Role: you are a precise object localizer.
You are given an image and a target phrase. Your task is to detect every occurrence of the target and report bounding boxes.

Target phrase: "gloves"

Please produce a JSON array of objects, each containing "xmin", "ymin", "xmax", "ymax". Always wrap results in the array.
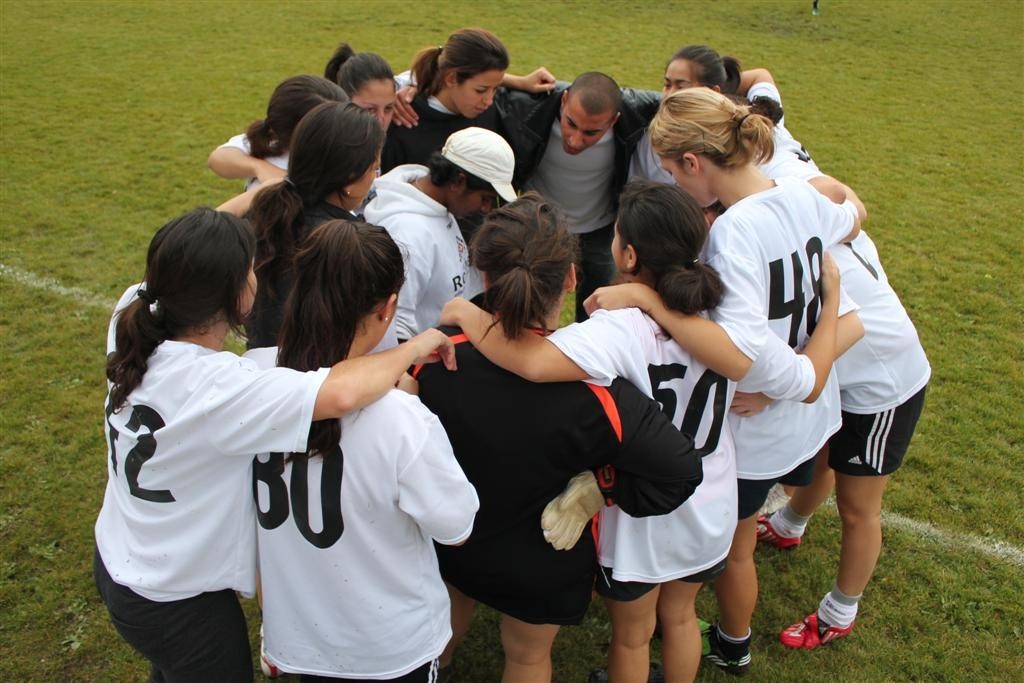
[{"xmin": 539, "ymin": 463, "xmax": 616, "ymax": 550}]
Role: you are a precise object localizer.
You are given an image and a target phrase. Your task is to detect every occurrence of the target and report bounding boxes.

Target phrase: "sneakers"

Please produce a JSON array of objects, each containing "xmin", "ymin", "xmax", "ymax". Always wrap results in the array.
[
  {"xmin": 259, "ymin": 619, "xmax": 286, "ymax": 678},
  {"xmin": 779, "ymin": 610, "xmax": 855, "ymax": 649},
  {"xmin": 756, "ymin": 514, "xmax": 800, "ymax": 549},
  {"xmin": 695, "ymin": 618, "xmax": 752, "ymax": 676},
  {"xmin": 758, "ymin": 483, "xmax": 791, "ymax": 515}
]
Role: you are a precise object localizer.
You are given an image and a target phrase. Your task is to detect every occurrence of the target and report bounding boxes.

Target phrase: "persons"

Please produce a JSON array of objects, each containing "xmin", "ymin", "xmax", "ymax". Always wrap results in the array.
[
  {"xmin": 92, "ymin": 178, "xmax": 457, "ymax": 683},
  {"xmin": 206, "ymin": 28, "xmax": 930, "ymax": 683}
]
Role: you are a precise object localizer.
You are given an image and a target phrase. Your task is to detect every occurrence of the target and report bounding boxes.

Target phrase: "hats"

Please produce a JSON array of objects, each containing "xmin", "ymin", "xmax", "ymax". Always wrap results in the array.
[{"xmin": 442, "ymin": 127, "xmax": 518, "ymax": 203}]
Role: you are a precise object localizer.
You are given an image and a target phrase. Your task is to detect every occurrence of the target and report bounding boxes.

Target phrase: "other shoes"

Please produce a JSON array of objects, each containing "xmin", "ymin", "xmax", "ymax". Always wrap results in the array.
[
  {"xmin": 589, "ymin": 664, "xmax": 609, "ymax": 683},
  {"xmin": 648, "ymin": 662, "xmax": 665, "ymax": 683}
]
